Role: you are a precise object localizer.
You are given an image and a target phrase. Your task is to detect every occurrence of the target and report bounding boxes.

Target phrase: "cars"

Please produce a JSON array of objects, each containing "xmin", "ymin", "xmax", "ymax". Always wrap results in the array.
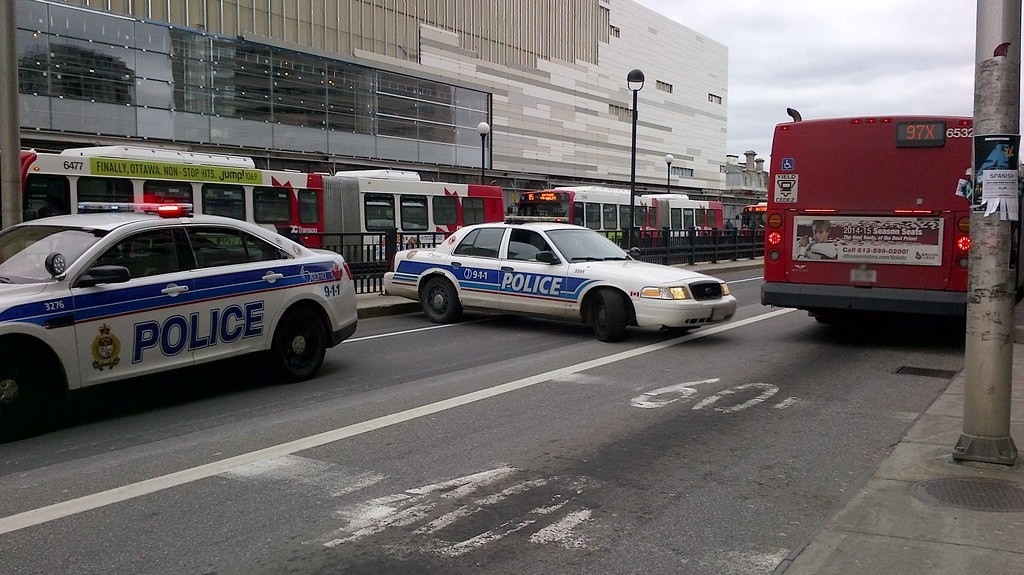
[
  {"xmin": 0, "ymin": 200, "xmax": 360, "ymax": 435},
  {"xmin": 384, "ymin": 216, "xmax": 738, "ymax": 342}
]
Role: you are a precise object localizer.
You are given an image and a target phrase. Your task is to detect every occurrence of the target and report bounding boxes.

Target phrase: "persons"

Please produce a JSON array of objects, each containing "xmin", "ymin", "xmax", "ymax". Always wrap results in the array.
[
  {"xmin": 750, "ymin": 218, "xmax": 761, "ymax": 229},
  {"xmin": 725, "ymin": 219, "xmax": 733, "ymax": 238},
  {"xmin": 797, "ymin": 219, "xmax": 837, "ymax": 260}
]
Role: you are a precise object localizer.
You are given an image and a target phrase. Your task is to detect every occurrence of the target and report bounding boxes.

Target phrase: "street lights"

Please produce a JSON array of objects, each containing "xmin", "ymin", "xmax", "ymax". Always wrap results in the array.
[
  {"xmin": 627, "ymin": 70, "xmax": 645, "ymax": 252},
  {"xmin": 663, "ymin": 153, "xmax": 673, "ymax": 194},
  {"xmin": 477, "ymin": 120, "xmax": 491, "ymax": 185}
]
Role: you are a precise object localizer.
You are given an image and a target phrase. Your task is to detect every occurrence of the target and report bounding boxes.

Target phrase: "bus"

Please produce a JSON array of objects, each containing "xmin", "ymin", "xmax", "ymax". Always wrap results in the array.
[
  {"xmin": 15, "ymin": 142, "xmax": 507, "ymax": 275},
  {"xmin": 736, "ymin": 202, "xmax": 767, "ymax": 246},
  {"xmin": 760, "ymin": 107, "xmax": 1024, "ymax": 332},
  {"xmin": 509, "ymin": 186, "xmax": 724, "ymax": 253}
]
